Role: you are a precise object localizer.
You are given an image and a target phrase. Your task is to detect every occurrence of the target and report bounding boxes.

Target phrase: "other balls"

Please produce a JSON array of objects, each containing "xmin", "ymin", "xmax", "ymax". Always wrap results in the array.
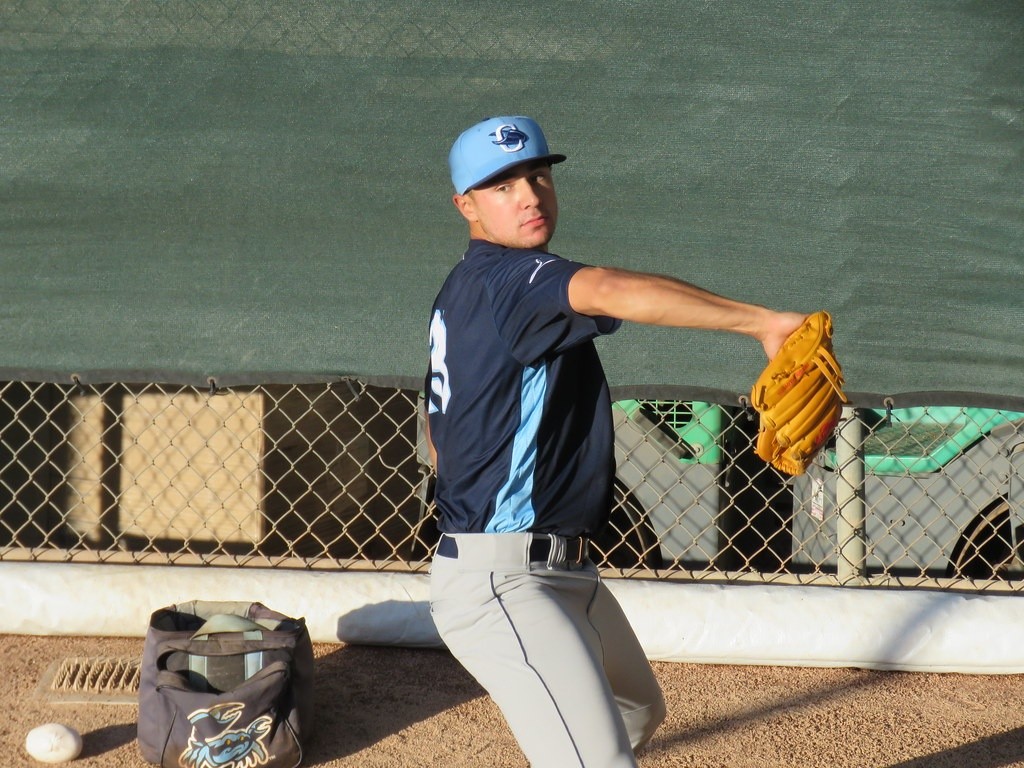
[{"xmin": 24, "ymin": 724, "xmax": 85, "ymax": 763}]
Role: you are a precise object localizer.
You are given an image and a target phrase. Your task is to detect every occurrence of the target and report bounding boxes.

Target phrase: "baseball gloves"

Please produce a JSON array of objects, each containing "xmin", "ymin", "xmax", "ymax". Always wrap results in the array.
[{"xmin": 748, "ymin": 309, "xmax": 848, "ymax": 475}]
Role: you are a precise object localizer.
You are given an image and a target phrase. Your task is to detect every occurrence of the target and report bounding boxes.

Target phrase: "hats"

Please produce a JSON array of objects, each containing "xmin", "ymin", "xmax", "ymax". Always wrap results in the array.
[{"xmin": 447, "ymin": 113, "xmax": 568, "ymax": 196}]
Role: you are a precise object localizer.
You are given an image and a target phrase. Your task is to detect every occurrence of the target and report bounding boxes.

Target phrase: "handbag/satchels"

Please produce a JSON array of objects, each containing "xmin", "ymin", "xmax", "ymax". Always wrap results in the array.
[{"xmin": 136, "ymin": 599, "xmax": 317, "ymax": 768}]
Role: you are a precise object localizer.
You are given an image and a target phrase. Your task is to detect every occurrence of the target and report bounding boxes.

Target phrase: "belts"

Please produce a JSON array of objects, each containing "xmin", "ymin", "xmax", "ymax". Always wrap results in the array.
[{"xmin": 437, "ymin": 533, "xmax": 590, "ymax": 563}]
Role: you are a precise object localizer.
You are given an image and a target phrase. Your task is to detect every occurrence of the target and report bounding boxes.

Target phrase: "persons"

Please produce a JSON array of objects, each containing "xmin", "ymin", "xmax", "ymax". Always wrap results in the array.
[{"xmin": 424, "ymin": 116, "xmax": 847, "ymax": 767}]
